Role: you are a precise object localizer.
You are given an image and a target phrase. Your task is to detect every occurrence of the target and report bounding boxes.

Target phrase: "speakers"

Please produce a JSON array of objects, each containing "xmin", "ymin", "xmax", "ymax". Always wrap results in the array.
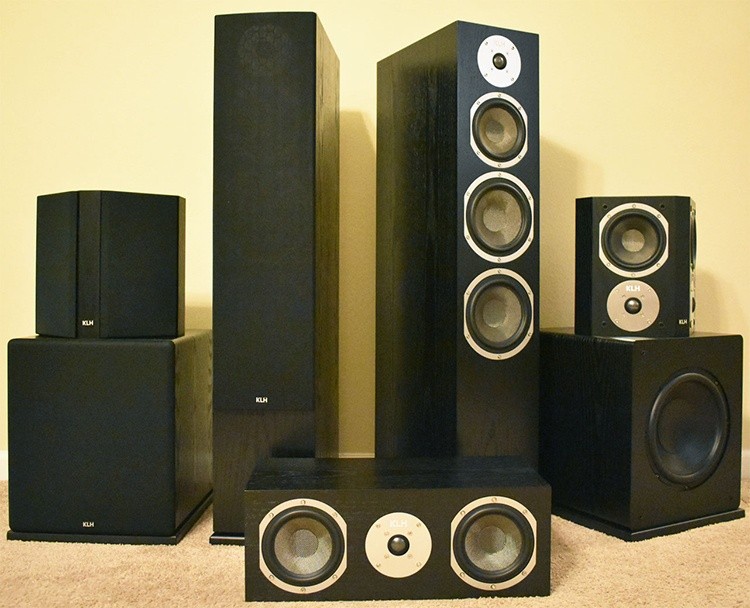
[
  {"xmin": 375, "ymin": 20, "xmax": 540, "ymax": 474},
  {"xmin": 210, "ymin": 10, "xmax": 341, "ymax": 548},
  {"xmin": 574, "ymin": 195, "xmax": 697, "ymax": 338},
  {"xmin": 540, "ymin": 327, "xmax": 745, "ymax": 543},
  {"xmin": 243, "ymin": 457, "xmax": 552, "ymax": 602},
  {"xmin": 7, "ymin": 328, "xmax": 213, "ymax": 545},
  {"xmin": 36, "ymin": 190, "xmax": 186, "ymax": 339}
]
